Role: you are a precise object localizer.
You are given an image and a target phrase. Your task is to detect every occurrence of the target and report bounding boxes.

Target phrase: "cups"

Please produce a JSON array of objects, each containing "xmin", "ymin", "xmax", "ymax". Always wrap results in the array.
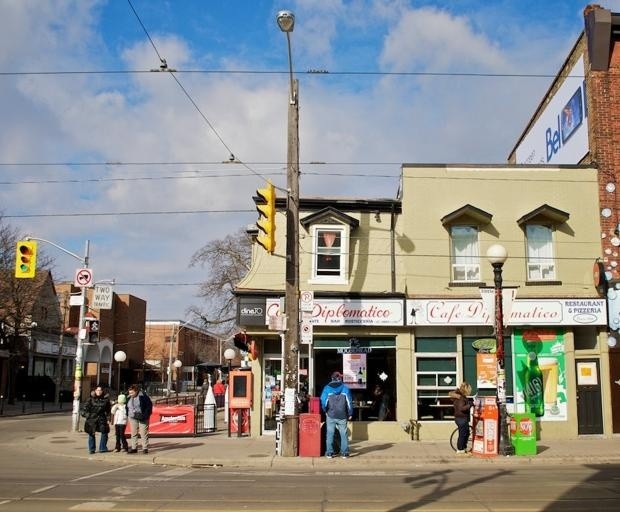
[{"xmin": 535, "ymin": 354, "xmax": 562, "ymax": 410}]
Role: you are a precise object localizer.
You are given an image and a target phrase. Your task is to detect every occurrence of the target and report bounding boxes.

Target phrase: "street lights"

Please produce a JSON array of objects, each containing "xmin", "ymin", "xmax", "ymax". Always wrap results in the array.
[
  {"xmin": 113, "ymin": 350, "xmax": 127, "ymax": 394},
  {"xmin": 487, "ymin": 245, "xmax": 515, "ymax": 456},
  {"xmin": 274, "ymin": 10, "xmax": 300, "ymax": 457},
  {"xmin": 167, "ymin": 315, "xmax": 198, "ymax": 397},
  {"xmin": 175, "ymin": 360, "xmax": 182, "ymax": 402},
  {"xmin": 224, "ymin": 348, "xmax": 235, "ymax": 372}
]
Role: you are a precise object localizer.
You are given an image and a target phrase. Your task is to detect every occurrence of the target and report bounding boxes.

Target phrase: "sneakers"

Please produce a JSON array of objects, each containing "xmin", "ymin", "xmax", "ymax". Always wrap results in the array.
[
  {"xmin": 457, "ymin": 450, "xmax": 471, "ymax": 456},
  {"xmin": 326, "ymin": 452, "xmax": 348, "ymax": 459},
  {"xmin": 114, "ymin": 449, "xmax": 148, "ymax": 454}
]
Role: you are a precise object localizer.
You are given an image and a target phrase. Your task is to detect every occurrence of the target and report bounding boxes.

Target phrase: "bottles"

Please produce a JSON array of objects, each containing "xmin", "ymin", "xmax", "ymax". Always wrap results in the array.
[
  {"xmin": 523, "ymin": 341, "xmax": 544, "ymax": 417},
  {"xmin": 204, "ymin": 381, "xmax": 217, "ymax": 433}
]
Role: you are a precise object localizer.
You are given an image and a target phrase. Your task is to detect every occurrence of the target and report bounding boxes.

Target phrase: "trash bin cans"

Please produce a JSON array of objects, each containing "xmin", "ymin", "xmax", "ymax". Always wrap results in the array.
[{"xmin": 280, "ymin": 413, "xmax": 299, "ymax": 459}]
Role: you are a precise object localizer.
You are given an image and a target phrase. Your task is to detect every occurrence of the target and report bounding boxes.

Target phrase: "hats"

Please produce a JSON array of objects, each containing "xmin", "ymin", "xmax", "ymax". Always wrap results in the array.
[
  {"xmin": 332, "ymin": 372, "xmax": 344, "ymax": 380},
  {"xmin": 118, "ymin": 394, "xmax": 127, "ymax": 403}
]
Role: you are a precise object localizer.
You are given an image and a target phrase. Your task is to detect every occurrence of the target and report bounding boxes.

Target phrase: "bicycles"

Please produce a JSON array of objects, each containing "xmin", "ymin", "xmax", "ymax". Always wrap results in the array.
[{"xmin": 451, "ymin": 424, "xmax": 515, "ymax": 454}]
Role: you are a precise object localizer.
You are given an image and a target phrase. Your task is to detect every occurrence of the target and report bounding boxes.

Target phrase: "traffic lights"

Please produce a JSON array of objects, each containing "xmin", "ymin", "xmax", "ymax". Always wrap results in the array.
[
  {"xmin": 90, "ymin": 321, "xmax": 100, "ymax": 344},
  {"xmin": 256, "ymin": 183, "xmax": 275, "ymax": 257},
  {"xmin": 15, "ymin": 240, "xmax": 36, "ymax": 279}
]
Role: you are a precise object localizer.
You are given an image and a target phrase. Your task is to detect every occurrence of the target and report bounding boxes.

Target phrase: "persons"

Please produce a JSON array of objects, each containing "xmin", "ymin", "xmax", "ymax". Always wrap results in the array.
[
  {"xmin": 213, "ymin": 379, "xmax": 225, "ymax": 397},
  {"xmin": 80, "ymin": 384, "xmax": 114, "ymax": 454},
  {"xmin": 85, "ymin": 388, "xmax": 96, "ymax": 403},
  {"xmin": 200, "ymin": 379, "xmax": 209, "ymax": 397},
  {"xmin": 453, "ymin": 381, "xmax": 478, "ymax": 455},
  {"xmin": 361, "ymin": 380, "xmax": 386, "ymax": 420},
  {"xmin": 320, "ymin": 371, "xmax": 355, "ymax": 458},
  {"xmin": 126, "ymin": 384, "xmax": 154, "ymax": 454},
  {"xmin": 110, "ymin": 394, "xmax": 130, "ymax": 453}
]
